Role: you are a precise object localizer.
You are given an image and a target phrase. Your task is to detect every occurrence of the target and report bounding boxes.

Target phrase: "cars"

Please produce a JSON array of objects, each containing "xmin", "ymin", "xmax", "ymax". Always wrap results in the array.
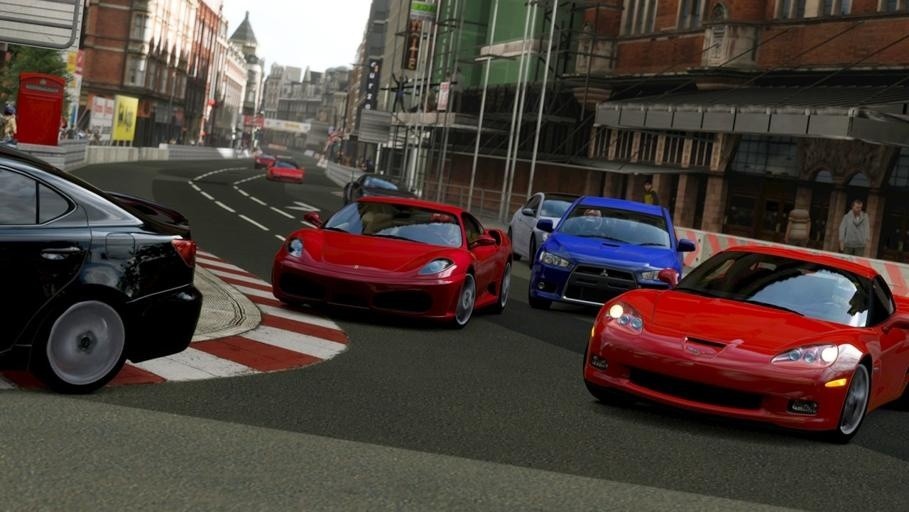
[
  {"xmin": 0, "ymin": 140, "xmax": 203, "ymax": 395},
  {"xmin": 508, "ymin": 192, "xmax": 697, "ymax": 311}
]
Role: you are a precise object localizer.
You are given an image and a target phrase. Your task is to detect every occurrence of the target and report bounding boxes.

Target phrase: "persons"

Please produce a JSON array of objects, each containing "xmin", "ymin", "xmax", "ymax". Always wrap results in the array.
[
  {"xmin": 642, "ymin": 181, "xmax": 659, "ymax": 206},
  {"xmin": 569, "ymin": 208, "xmax": 608, "ymax": 234},
  {"xmin": 784, "ymin": 197, "xmax": 812, "ymax": 245},
  {"xmin": 59, "ymin": 125, "xmax": 91, "ymax": 141},
  {"xmin": 838, "ymin": 197, "xmax": 870, "ymax": 257},
  {"xmin": 0, "ymin": 104, "xmax": 17, "ymax": 145},
  {"xmin": 392, "ymin": 72, "xmax": 408, "ymax": 112}
]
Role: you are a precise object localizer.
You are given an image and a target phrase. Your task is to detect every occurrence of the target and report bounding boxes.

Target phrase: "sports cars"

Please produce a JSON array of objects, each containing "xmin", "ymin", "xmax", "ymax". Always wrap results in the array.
[
  {"xmin": 583, "ymin": 245, "xmax": 909, "ymax": 445},
  {"xmin": 271, "ymin": 173, "xmax": 514, "ymax": 330},
  {"xmin": 253, "ymin": 151, "xmax": 306, "ymax": 183}
]
